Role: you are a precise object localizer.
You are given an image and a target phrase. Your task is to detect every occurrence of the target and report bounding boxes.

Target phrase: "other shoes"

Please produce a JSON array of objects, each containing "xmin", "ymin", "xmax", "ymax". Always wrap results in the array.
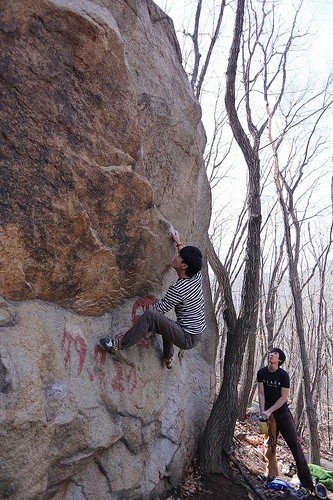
[
  {"xmin": 99, "ymin": 337, "xmax": 119, "ymax": 357},
  {"xmin": 298, "ymin": 486, "xmax": 312, "ymax": 497},
  {"xmin": 165, "ymin": 357, "xmax": 173, "ymax": 369}
]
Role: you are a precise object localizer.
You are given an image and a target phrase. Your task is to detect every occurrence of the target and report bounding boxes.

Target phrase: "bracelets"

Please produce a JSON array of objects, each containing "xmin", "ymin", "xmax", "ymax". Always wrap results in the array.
[{"xmin": 176, "ymin": 244, "xmax": 184, "ymax": 247}]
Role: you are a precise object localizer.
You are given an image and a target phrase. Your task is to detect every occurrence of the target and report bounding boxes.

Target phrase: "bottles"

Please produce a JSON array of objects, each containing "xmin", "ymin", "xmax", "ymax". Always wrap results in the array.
[{"xmin": 258, "ymin": 415, "xmax": 269, "ymax": 434}]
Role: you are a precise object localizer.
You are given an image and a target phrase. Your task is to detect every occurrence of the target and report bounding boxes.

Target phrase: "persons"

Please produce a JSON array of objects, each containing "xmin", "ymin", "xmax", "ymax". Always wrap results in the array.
[
  {"xmin": 258, "ymin": 348, "xmax": 314, "ymax": 489},
  {"xmin": 98, "ymin": 230, "xmax": 207, "ymax": 370}
]
